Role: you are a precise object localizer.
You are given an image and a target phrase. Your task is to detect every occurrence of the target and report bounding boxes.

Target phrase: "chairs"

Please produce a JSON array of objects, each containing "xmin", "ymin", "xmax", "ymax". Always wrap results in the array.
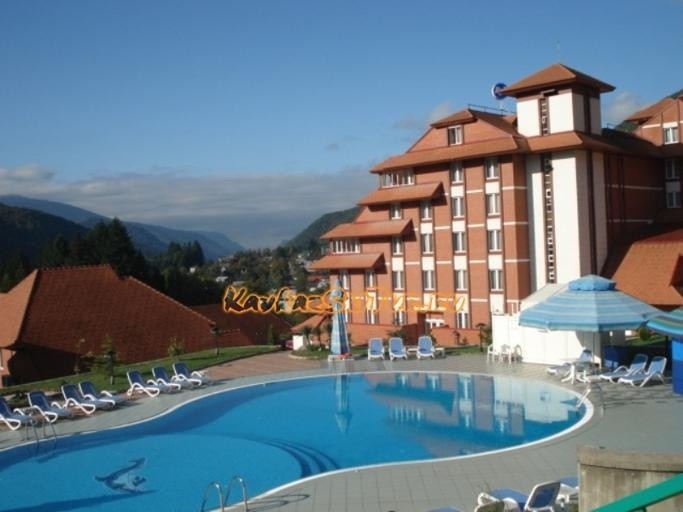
[
  {"xmin": 546, "ymin": 349, "xmax": 668, "ymax": 388},
  {"xmin": 426, "ymin": 475, "xmax": 580, "ymax": 512},
  {"xmin": 487, "ymin": 342, "xmax": 522, "ymax": 364},
  {"xmin": 0, "ymin": 362, "xmax": 212, "ymax": 434},
  {"xmin": 365, "ymin": 335, "xmax": 436, "ymax": 362}
]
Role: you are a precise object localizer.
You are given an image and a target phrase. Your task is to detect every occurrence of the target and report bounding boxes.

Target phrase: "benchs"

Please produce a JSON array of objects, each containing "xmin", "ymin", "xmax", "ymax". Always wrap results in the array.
[{"xmin": 406, "ymin": 348, "xmax": 445, "ymax": 356}]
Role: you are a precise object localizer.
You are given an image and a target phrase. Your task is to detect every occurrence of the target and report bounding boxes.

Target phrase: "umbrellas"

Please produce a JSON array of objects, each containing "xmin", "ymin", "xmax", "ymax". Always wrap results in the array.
[
  {"xmin": 332, "ymin": 375, "xmax": 353, "ymax": 438},
  {"xmin": 515, "ymin": 273, "xmax": 659, "ymax": 369},
  {"xmin": 329, "ymin": 280, "xmax": 351, "ymax": 358},
  {"xmin": 644, "ymin": 303, "xmax": 683, "ymax": 341}
]
